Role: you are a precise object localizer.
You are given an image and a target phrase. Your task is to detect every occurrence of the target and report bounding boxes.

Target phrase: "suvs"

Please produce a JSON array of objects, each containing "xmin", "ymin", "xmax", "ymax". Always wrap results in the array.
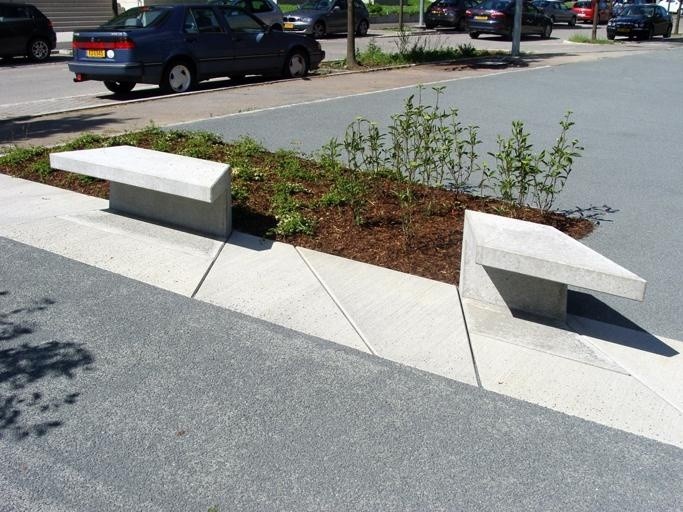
[{"xmin": 68, "ymin": 1, "xmax": 325, "ymax": 94}]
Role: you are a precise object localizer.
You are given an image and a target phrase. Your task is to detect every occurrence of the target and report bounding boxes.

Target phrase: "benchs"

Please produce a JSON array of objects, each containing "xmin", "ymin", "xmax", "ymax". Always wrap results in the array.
[
  {"xmin": 456, "ymin": 206, "xmax": 647, "ymax": 328},
  {"xmin": 46, "ymin": 144, "xmax": 234, "ymax": 239}
]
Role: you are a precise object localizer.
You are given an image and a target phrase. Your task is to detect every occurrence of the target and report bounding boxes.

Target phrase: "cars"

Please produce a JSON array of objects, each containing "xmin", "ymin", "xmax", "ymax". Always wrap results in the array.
[
  {"xmin": 424, "ymin": 0, "xmax": 683, "ymax": 41},
  {"xmin": 0, "ymin": 1, "xmax": 59, "ymax": 63},
  {"xmin": 282, "ymin": 0, "xmax": 369, "ymax": 39},
  {"xmin": 184, "ymin": 1, "xmax": 284, "ymax": 33}
]
